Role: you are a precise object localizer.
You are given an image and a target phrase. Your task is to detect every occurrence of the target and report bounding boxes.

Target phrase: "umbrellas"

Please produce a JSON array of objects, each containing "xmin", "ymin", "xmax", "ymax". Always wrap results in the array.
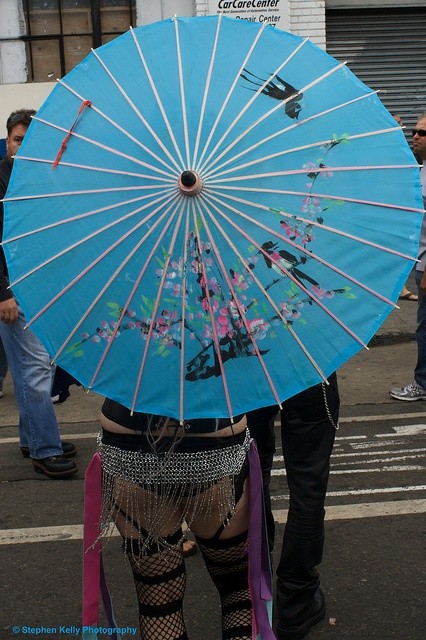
[{"xmin": 0, "ymin": 14, "xmax": 426, "ymax": 426}]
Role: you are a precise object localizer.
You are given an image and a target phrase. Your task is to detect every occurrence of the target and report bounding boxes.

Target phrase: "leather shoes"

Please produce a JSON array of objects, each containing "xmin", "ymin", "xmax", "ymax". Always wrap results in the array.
[
  {"xmin": 22, "ymin": 442, "xmax": 75, "ymax": 456},
  {"xmin": 275, "ymin": 588, "xmax": 325, "ymax": 640}
]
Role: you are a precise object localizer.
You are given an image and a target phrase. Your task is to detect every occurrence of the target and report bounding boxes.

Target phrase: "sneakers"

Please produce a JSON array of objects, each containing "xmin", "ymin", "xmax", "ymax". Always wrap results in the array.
[
  {"xmin": 33, "ymin": 457, "xmax": 76, "ymax": 474},
  {"xmin": 390, "ymin": 380, "xmax": 426, "ymax": 401}
]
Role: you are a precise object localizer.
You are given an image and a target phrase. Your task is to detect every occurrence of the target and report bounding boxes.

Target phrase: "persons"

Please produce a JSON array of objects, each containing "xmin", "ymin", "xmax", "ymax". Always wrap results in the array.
[
  {"xmin": 2, "ymin": 109, "xmax": 77, "ymax": 476},
  {"xmin": 389, "ymin": 115, "xmax": 426, "ymax": 401},
  {"xmin": 248, "ymin": 372, "xmax": 339, "ymax": 640},
  {"xmin": 94, "ymin": 397, "xmax": 261, "ymax": 640}
]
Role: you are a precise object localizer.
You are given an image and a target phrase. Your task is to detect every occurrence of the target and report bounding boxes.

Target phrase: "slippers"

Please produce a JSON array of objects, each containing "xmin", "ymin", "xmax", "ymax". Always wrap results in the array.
[{"xmin": 399, "ymin": 292, "xmax": 418, "ymax": 301}]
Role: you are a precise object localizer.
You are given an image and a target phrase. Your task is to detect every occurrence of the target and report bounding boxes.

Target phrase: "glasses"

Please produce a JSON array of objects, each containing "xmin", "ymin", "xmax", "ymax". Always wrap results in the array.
[{"xmin": 412, "ymin": 130, "xmax": 426, "ymax": 136}]
